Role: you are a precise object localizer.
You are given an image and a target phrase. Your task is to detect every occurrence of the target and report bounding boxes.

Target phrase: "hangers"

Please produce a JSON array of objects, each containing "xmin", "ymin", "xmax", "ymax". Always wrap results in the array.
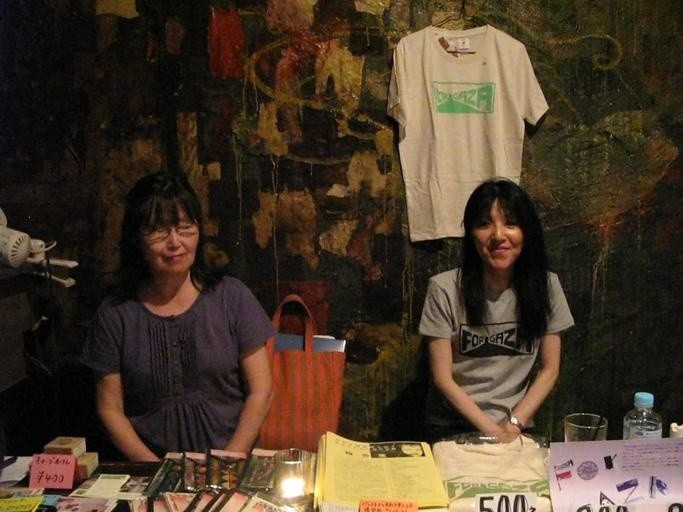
[{"xmin": 395, "ymin": 1, "xmax": 525, "ymax": 56}]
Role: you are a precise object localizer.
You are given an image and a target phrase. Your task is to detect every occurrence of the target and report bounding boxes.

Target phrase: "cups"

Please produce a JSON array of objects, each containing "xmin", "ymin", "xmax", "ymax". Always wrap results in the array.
[
  {"xmin": 272, "ymin": 447, "xmax": 312, "ymax": 511},
  {"xmin": 564, "ymin": 413, "xmax": 608, "ymax": 443}
]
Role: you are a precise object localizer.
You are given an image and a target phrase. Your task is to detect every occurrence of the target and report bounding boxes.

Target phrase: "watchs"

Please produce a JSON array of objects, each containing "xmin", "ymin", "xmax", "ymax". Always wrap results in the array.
[{"xmin": 506, "ymin": 415, "xmax": 525, "ymax": 432}]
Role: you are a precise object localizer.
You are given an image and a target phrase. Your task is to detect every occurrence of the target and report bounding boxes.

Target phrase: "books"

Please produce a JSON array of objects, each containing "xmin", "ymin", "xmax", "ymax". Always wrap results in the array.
[{"xmin": 310, "ymin": 430, "xmax": 450, "ymax": 512}]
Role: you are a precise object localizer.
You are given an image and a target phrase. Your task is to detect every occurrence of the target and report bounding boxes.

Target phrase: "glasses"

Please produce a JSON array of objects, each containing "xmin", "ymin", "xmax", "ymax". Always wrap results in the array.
[{"xmin": 140, "ymin": 221, "xmax": 198, "ymax": 245}]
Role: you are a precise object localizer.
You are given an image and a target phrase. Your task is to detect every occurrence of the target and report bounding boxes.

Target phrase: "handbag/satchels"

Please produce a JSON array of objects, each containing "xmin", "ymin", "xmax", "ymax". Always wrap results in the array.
[{"xmin": 259, "ymin": 294, "xmax": 347, "ymax": 453}]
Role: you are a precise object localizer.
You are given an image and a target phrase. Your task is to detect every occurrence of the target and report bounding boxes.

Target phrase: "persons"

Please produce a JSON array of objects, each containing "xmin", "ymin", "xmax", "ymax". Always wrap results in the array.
[
  {"xmin": 84, "ymin": 168, "xmax": 275, "ymax": 463},
  {"xmin": 414, "ymin": 177, "xmax": 577, "ymax": 443}
]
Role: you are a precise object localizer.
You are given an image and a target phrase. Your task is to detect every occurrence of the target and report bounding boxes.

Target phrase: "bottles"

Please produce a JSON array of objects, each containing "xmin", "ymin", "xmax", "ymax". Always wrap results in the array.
[{"xmin": 623, "ymin": 392, "xmax": 663, "ymax": 440}]
